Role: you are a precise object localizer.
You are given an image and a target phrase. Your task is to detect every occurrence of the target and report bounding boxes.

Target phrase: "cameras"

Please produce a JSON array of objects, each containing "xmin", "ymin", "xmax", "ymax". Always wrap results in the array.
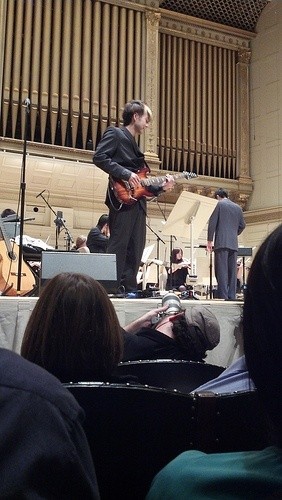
[{"xmin": 150, "ymin": 293, "xmax": 182, "ymax": 325}]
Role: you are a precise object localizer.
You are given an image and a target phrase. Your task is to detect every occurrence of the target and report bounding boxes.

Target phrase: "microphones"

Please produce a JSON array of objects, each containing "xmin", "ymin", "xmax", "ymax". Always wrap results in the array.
[
  {"xmin": 24, "ymin": 98, "xmax": 31, "ymax": 113},
  {"xmin": 56, "ymin": 211, "xmax": 63, "ymax": 235},
  {"xmin": 149, "ymin": 197, "xmax": 157, "ymax": 203},
  {"xmin": 36, "ymin": 190, "xmax": 45, "ymax": 198}
]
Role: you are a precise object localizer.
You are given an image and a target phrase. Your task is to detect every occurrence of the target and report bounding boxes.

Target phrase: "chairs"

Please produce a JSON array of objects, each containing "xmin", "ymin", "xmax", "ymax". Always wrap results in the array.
[{"xmin": 62, "ymin": 359, "xmax": 275, "ymax": 500}]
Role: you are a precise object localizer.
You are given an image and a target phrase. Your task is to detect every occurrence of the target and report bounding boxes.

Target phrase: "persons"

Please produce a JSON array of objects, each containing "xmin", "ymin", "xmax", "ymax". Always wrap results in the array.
[
  {"xmin": 207, "ymin": 189, "xmax": 246, "ymax": 299},
  {"xmin": 0, "ymin": 209, "xmax": 282, "ymax": 500},
  {"xmin": 93, "ymin": 100, "xmax": 174, "ymax": 298}
]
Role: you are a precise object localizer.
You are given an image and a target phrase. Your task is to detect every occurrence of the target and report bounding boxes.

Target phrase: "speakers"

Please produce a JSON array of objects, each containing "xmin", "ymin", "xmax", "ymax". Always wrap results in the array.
[{"xmin": 39, "ymin": 251, "xmax": 118, "ymax": 295}]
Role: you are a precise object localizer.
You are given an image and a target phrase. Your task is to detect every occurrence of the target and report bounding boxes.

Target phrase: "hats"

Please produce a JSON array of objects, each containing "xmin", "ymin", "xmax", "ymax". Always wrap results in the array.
[{"xmin": 184, "ymin": 305, "xmax": 221, "ymax": 351}]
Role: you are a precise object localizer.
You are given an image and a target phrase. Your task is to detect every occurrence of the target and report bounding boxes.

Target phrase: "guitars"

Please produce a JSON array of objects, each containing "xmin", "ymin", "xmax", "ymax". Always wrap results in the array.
[
  {"xmin": 108, "ymin": 167, "xmax": 198, "ymax": 208},
  {"xmin": 0, "ymin": 215, "xmax": 36, "ymax": 296}
]
